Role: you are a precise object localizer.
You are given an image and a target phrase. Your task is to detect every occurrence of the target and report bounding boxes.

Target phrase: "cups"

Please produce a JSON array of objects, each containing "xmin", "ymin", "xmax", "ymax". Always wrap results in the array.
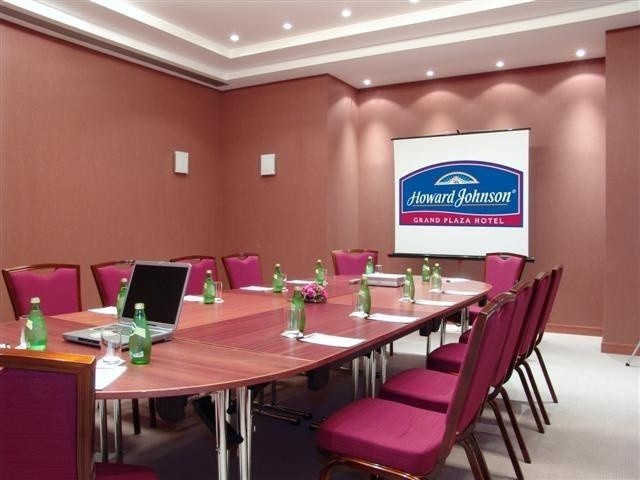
[
  {"xmin": 323, "ymin": 268, "xmax": 328, "ymax": 285},
  {"xmin": 281, "ymin": 274, "xmax": 287, "ymax": 291},
  {"xmin": 400, "ymin": 280, "xmax": 412, "ymax": 304},
  {"xmin": 375, "ymin": 264, "xmax": 383, "ymax": 275},
  {"xmin": 429, "ymin": 276, "xmax": 442, "ymax": 292},
  {"xmin": 286, "ymin": 304, "xmax": 300, "ymax": 335},
  {"xmin": 214, "ymin": 282, "xmax": 223, "ymax": 301},
  {"xmin": 101, "ymin": 328, "xmax": 123, "ymax": 365},
  {"xmin": 352, "ymin": 290, "xmax": 365, "ymax": 315},
  {"xmin": 18, "ymin": 315, "xmax": 28, "ymax": 348}
]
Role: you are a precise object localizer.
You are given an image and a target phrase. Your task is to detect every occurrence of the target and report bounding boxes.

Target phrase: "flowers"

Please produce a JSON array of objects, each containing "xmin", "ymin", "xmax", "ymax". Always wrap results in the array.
[{"xmin": 300, "ymin": 282, "xmax": 329, "ymax": 303}]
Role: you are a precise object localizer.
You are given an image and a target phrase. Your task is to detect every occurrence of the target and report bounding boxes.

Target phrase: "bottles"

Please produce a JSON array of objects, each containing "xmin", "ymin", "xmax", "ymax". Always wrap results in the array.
[
  {"xmin": 272, "ymin": 264, "xmax": 282, "ymax": 293},
  {"xmin": 291, "ymin": 286, "xmax": 305, "ymax": 333},
  {"xmin": 203, "ymin": 270, "xmax": 215, "ymax": 304},
  {"xmin": 25, "ymin": 297, "xmax": 47, "ymax": 352},
  {"xmin": 117, "ymin": 277, "xmax": 128, "ymax": 318},
  {"xmin": 315, "ymin": 259, "xmax": 323, "ymax": 285},
  {"xmin": 405, "ymin": 268, "xmax": 415, "ymax": 303},
  {"xmin": 432, "ymin": 262, "xmax": 441, "ymax": 289},
  {"xmin": 422, "ymin": 257, "xmax": 430, "ymax": 283},
  {"xmin": 365, "ymin": 255, "xmax": 374, "ymax": 274},
  {"xmin": 128, "ymin": 302, "xmax": 152, "ymax": 364},
  {"xmin": 358, "ymin": 274, "xmax": 371, "ymax": 315}
]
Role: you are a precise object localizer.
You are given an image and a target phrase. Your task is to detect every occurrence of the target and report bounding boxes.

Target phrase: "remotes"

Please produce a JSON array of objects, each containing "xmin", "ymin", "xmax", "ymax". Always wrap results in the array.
[{"xmin": 349, "ymin": 279, "xmax": 361, "ymax": 284}]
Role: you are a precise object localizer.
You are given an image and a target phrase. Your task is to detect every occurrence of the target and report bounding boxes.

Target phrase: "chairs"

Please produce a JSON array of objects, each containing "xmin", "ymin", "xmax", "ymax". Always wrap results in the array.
[
  {"xmin": 438, "ymin": 252, "xmax": 527, "ymax": 346},
  {"xmin": 424, "ymin": 272, "xmax": 553, "ymax": 463},
  {"xmin": 316, "ymin": 292, "xmax": 517, "ymax": 480},
  {"xmin": 331, "ymin": 248, "xmax": 380, "ymax": 274},
  {"xmin": 221, "ymin": 251, "xmax": 264, "ymax": 289},
  {"xmin": 2, "ymin": 262, "xmax": 126, "ymax": 466},
  {"xmin": 170, "ymin": 255, "xmax": 218, "ymax": 295},
  {"xmin": 380, "ymin": 278, "xmax": 536, "ymax": 479},
  {"xmin": 90, "ymin": 258, "xmax": 164, "ymax": 434},
  {"xmin": 460, "ymin": 265, "xmax": 564, "ymax": 424},
  {"xmin": 0, "ymin": 349, "xmax": 158, "ymax": 480}
]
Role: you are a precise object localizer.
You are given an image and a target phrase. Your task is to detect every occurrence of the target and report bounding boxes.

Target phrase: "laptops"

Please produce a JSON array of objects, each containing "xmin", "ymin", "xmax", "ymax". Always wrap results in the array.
[{"xmin": 62, "ymin": 260, "xmax": 192, "ymax": 353}]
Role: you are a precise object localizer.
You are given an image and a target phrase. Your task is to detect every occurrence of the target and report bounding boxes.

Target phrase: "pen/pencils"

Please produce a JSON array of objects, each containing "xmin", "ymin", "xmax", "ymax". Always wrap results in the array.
[{"xmin": 298, "ymin": 334, "xmax": 313, "ymax": 339}]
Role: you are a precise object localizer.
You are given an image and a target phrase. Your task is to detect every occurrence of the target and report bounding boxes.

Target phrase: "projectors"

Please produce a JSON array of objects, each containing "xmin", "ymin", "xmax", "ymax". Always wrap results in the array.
[{"xmin": 366, "ymin": 272, "xmax": 407, "ymax": 288}]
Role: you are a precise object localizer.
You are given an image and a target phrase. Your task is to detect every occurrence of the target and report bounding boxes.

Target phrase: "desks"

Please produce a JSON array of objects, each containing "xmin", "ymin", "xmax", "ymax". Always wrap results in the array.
[{"xmin": 0, "ymin": 276, "xmax": 492, "ymax": 480}]
